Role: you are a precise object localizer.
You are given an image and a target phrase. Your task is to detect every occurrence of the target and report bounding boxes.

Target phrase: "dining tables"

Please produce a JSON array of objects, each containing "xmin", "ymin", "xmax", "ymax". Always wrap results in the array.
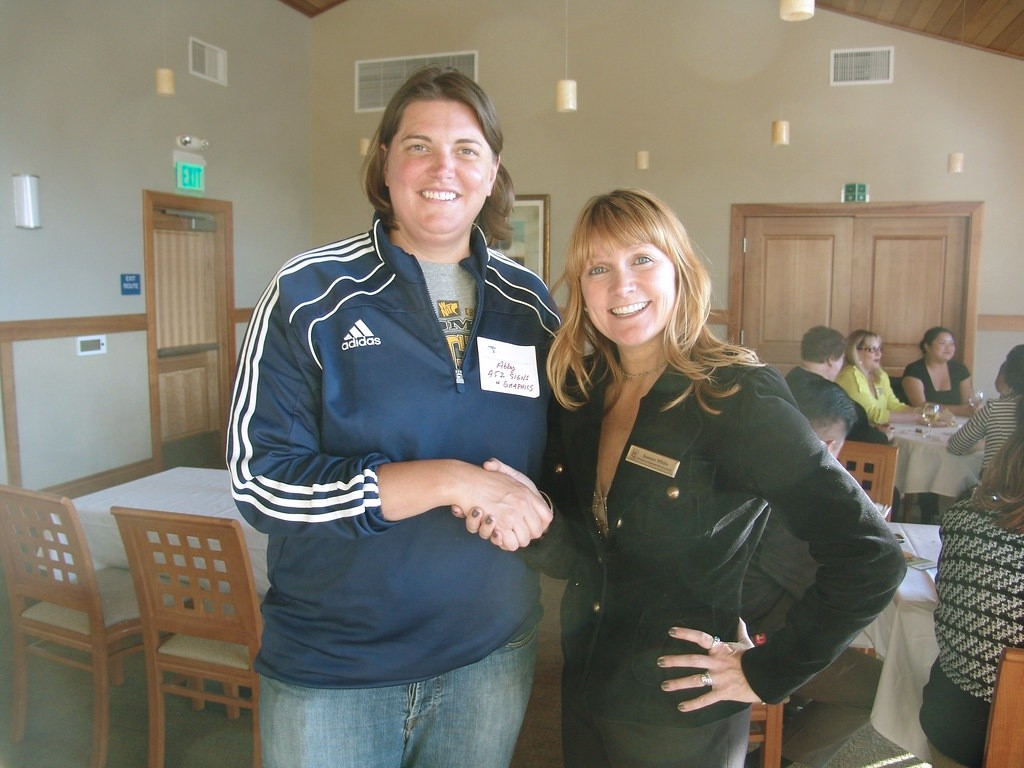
[
  {"xmin": 880, "ymin": 414, "xmax": 987, "ymax": 522},
  {"xmin": 36, "ymin": 466, "xmax": 273, "ymax": 597},
  {"xmin": 826, "ymin": 523, "xmax": 942, "ymax": 760}
]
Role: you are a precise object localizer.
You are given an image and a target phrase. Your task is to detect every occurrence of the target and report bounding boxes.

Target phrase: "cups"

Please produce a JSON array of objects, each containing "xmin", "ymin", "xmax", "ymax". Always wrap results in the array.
[{"xmin": 969, "ymin": 390, "xmax": 984, "ymax": 409}]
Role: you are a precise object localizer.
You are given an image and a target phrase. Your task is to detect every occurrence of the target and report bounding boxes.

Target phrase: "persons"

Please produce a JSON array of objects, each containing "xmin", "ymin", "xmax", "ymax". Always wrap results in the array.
[
  {"xmin": 741, "ymin": 366, "xmax": 885, "ymax": 768},
  {"xmin": 901, "ymin": 327, "xmax": 974, "ymax": 524},
  {"xmin": 785, "ymin": 326, "xmax": 899, "ymax": 521},
  {"xmin": 918, "ymin": 398, "xmax": 1024, "ymax": 768},
  {"xmin": 835, "ymin": 330, "xmax": 954, "ymax": 427},
  {"xmin": 225, "ymin": 62, "xmax": 560, "ymax": 768},
  {"xmin": 949, "ymin": 344, "xmax": 1024, "ymax": 502},
  {"xmin": 453, "ymin": 189, "xmax": 907, "ymax": 768}
]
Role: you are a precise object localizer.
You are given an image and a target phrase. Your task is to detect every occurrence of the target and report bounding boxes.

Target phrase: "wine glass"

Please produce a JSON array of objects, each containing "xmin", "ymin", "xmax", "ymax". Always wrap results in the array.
[{"xmin": 922, "ymin": 402, "xmax": 940, "ymax": 440}]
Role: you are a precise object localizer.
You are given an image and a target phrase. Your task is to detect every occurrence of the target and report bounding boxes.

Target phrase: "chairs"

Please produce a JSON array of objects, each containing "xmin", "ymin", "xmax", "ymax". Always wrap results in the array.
[
  {"xmin": 110, "ymin": 504, "xmax": 264, "ymax": 768},
  {"xmin": 746, "ymin": 439, "xmax": 1024, "ymax": 768},
  {"xmin": 0, "ymin": 485, "xmax": 206, "ymax": 768}
]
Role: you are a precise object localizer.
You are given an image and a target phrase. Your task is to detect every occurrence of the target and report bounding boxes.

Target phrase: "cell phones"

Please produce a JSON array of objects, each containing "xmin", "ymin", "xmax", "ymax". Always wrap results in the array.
[{"xmin": 895, "ymin": 534, "xmax": 906, "ymax": 543}]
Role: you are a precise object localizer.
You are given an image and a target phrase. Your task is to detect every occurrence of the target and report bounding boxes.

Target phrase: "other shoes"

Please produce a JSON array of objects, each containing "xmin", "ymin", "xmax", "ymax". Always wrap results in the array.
[{"xmin": 743, "ymin": 746, "xmax": 814, "ymax": 768}]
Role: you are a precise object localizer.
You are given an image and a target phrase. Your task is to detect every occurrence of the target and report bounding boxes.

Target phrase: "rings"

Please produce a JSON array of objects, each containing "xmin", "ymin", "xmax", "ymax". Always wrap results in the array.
[
  {"xmin": 701, "ymin": 672, "xmax": 712, "ymax": 686},
  {"xmin": 711, "ymin": 636, "xmax": 720, "ymax": 647}
]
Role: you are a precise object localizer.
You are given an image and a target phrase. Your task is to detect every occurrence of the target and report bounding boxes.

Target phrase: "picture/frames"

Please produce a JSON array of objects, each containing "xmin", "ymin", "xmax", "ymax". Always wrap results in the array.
[{"xmin": 489, "ymin": 193, "xmax": 550, "ymax": 290}]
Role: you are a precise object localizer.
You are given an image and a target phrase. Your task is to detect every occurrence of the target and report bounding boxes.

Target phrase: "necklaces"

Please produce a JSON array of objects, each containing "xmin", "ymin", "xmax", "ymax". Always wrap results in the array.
[{"xmin": 620, "ymin": 360, "xmax": 670, "ymax": 379}]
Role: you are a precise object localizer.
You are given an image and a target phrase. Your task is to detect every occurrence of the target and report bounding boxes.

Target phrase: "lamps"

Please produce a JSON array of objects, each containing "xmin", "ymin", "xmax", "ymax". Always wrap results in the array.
[
  {"xmin": 555, "ymin": 0, "xmax": 576, "ymax": 112},
  {"xmin": 12, "ymin": 174, "xmax": 42, "ymax": 227},
  {"xmin": 779, "ymin": 0, "xmax": 815, "ymax": 21},
  {"xmin": 178, "ymin": 136, "xmax": 210, "ymax": 151},
  {"xmin": 638, "ymin": 150, "xmax": 649, "ymax": 169},
  {"xmin": 772, "ymin": 81, "xmax": 790, "ymax": 144},
  {"xmin": 156, "ymin": 0, "xmax": 174, "ymax": 96},
  {"xmin": 949, "ymin": 0, "xmax": 965, "ymax": 174},
  {"xmin": 359, "ymin": 139, "xmax": 370, "ymax": 156}
]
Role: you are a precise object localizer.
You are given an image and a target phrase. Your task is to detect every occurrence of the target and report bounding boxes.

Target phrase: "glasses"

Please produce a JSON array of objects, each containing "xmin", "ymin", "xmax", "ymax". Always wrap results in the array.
[{"xmin": 857, "ymin": 347, "xmax": 882, "ymax": 353}]
